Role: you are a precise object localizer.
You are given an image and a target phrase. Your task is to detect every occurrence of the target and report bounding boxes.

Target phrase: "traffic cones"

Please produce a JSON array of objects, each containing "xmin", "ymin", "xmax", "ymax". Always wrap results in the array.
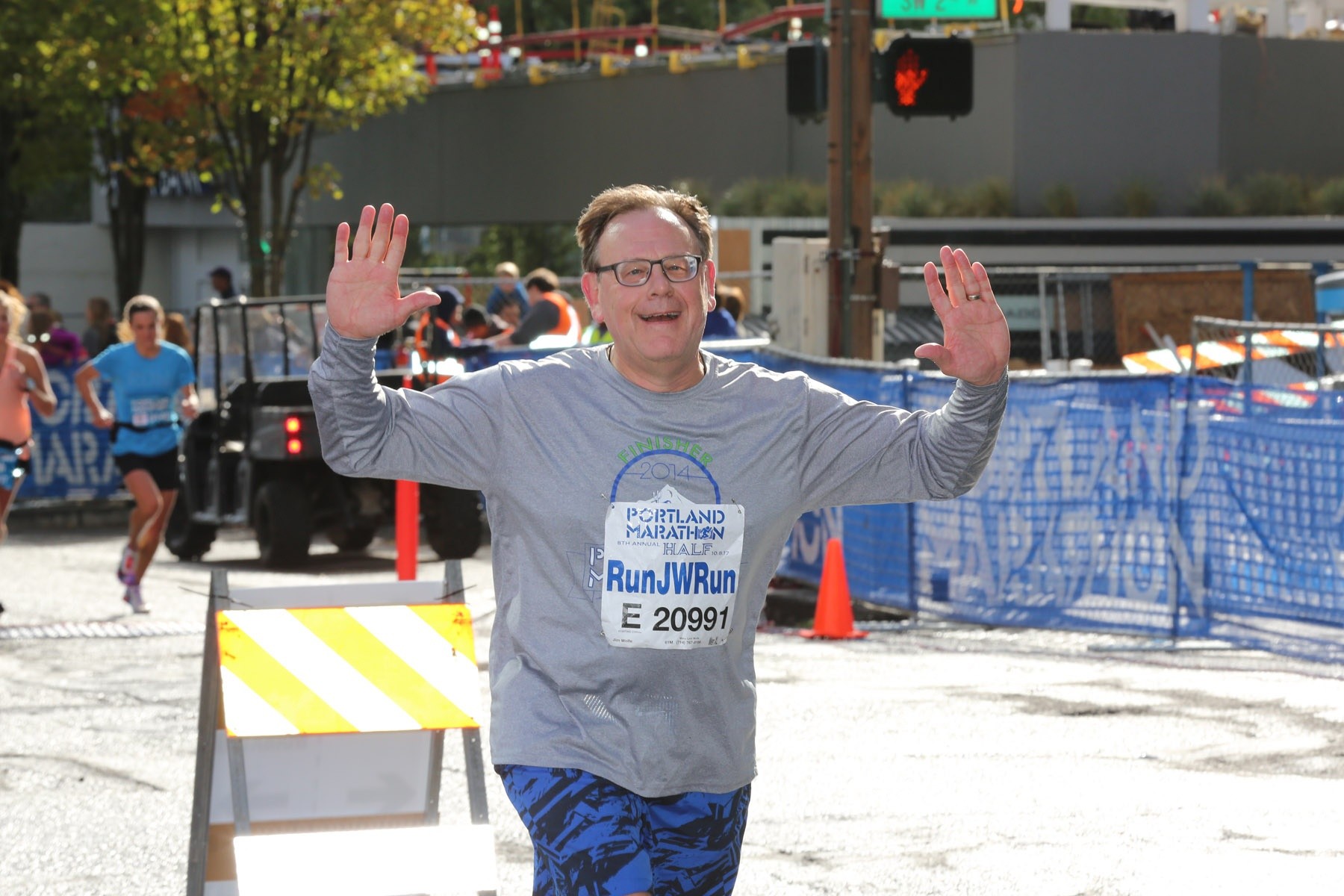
[{"xmin": 798, "ymin": 539, "xmax": 871, "ymax": 641}]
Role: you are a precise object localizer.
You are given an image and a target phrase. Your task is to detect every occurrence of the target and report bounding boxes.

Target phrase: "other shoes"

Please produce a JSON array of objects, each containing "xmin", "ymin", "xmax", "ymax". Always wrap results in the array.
[
  {"xmin": 123, "ymin": 584, "xmax": 151, "ymax": 613},
  {"xmin": 117, "ymin": 547, "xmax": 140, "ymax": 585}
]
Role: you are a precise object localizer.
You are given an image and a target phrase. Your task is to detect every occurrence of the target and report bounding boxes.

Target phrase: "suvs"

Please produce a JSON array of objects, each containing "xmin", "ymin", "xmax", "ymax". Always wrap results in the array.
[{"xmin": 163, "ymin": 295, "xmax": 483, "ymax": 568}]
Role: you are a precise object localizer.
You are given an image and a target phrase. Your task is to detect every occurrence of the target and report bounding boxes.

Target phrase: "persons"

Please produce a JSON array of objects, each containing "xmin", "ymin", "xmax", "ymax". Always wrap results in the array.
[
  {"xmin": 0, "ymin": 266, "xmax": 266, "ymax": 616},
  {"xmin": 308, "ymin": 184, "xmax": 1010, "ymax": 896},
  {"xmin": 398, "ymin": 262, "xmax": 747, "ymax": 395}
]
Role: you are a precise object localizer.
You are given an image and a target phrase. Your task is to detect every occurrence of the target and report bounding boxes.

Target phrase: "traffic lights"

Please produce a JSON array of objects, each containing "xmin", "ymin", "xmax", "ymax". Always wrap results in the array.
[
  {"xmin": 782, "ymin": 38, "xmax": 831, "ymax": 124},
  {"xmin": 1006, "ymin": 0, "xmax": 1046, "ymax": 18},
  {"xmin": 881, "ymin": 31, "xmax": 975, "ymax": 123}
]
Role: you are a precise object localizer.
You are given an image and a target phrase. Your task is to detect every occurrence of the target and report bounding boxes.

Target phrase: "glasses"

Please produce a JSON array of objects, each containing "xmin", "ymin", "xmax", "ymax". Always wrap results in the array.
[{"xmin": 596, "ymin": 254, "xmax": 702, "ymax": 286}]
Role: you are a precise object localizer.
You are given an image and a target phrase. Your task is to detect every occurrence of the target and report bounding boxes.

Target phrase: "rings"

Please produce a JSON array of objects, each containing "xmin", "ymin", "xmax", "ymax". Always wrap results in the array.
[{"xmin": 966, "ymin": 293, "xmax": 981, "ymax": 300}]
